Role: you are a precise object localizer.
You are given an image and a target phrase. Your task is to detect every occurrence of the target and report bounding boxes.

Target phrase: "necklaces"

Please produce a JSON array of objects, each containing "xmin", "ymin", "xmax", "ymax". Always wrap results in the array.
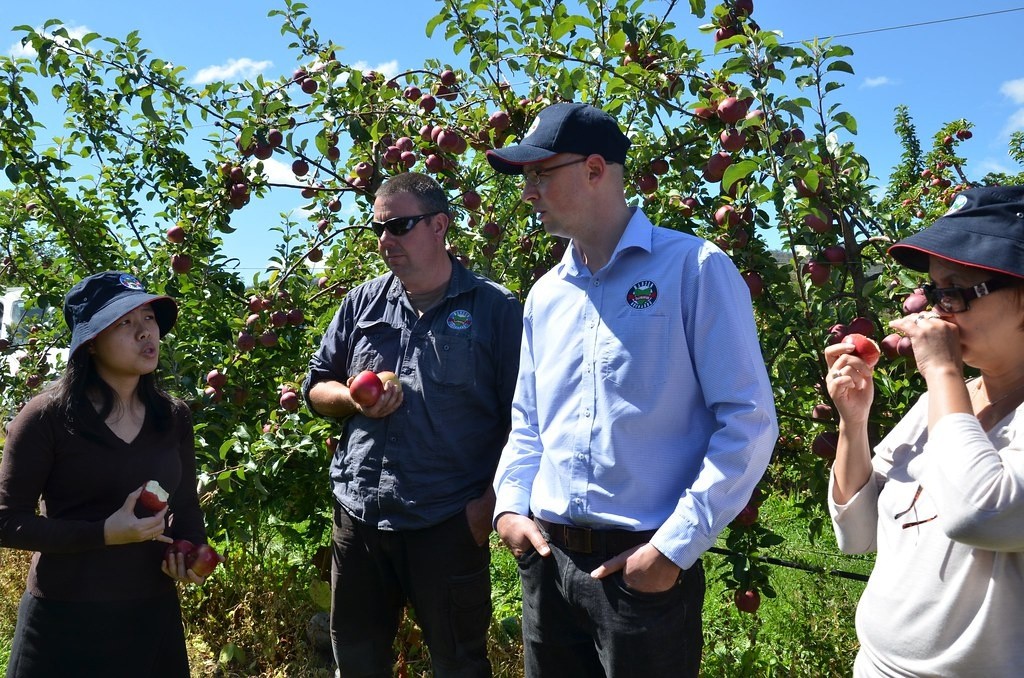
[{"xmin": 981, "ymin": 383, "xmax": 1024, "ymax": 406}]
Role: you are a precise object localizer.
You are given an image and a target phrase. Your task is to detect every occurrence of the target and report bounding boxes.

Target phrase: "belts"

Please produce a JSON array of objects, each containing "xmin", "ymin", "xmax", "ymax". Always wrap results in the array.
[{"xmin": 532, "ymin": 516, "xmax": 658, "ymax": 556}]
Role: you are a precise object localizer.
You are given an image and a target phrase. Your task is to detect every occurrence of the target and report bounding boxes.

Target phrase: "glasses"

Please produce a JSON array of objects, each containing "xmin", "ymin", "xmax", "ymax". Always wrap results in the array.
[
  {"xmin": 522, "ymin": 157, "xmax": 614, "ymax": 186},
  {"xmin": 923, "ymin": 274, "xmax": 1015, "ymax": 313},
  {"xmin": 371, "ymin": 211, "xmax": 445, "ymax": 236}
]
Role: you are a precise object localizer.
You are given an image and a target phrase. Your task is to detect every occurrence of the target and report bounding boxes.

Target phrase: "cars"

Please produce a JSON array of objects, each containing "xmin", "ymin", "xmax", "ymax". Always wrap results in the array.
[{"xmin": 0, "ymin": 287, "xmax": 70, "ymax": 435}]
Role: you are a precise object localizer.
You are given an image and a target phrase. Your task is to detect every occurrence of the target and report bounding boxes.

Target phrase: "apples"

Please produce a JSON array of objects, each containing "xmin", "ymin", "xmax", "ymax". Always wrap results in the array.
[{"xmin": 0, "ymin": 0, "xmax": 973, "ymax": 612}]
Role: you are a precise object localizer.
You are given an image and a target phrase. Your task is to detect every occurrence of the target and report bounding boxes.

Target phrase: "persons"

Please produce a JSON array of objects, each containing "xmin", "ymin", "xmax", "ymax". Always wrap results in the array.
[
  {"xmin": 0, "ymin": 271, "xmax": 226, "ymax": 678},
  {"xmin": 486, "ymin": 102, "xmax": 779, "ymax": 678},
  {"xmin": 825, "ymin": 185, "xmax": 1024, "ymax": 677},
  {"xmin": 302, "ymin": 173, "xmax": 525, "ymax": 678}
]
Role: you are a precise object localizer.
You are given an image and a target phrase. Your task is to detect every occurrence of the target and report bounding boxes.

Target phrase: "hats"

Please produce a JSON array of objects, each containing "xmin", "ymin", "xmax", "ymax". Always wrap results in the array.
[
  {"xmin": 62, "ymin": 271, "xmax": 178, "ymax": 369},
  {"xmin": 485, "ymin": 102, "xmax": 632, "ymax": 176},
  {"xmin": 888, "ymin": 185, "xmax": 1024, "ymax": 292}
]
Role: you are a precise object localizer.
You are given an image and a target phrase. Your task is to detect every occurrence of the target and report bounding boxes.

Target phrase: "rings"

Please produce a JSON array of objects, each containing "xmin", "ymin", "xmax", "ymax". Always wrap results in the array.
[
  {"xmin": 153, "ymin": 533, "xmax": 154, "ymax": 538},
  {"xmin": 832, "ymin": 370, "xmax": 843, "ymax": 379},
  {"xmin": 925, "ymin": 311, "xmax": 940, "ymax": 319},
  {"xmin": 152, "ymin": 538, "xmax": 155, "ymax": 540},
  {"xmin": 914, "ymin": 314, "xmax": 924, "ymax": 325}
]
